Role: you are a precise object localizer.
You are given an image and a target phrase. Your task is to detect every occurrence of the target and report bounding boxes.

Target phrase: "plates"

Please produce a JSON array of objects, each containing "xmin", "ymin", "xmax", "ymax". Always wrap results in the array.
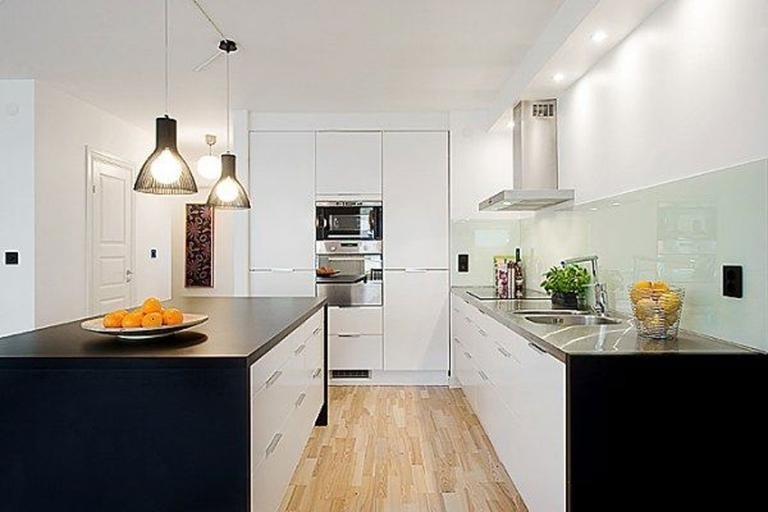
[{"xmin": 78, "ymin": 313, "xmax": 211, "ymax": 342}]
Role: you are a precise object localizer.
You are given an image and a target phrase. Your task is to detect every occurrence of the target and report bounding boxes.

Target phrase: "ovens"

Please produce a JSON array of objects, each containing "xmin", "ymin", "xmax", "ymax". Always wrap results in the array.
[{"xmin": 314, "ymin": 200, "xmax": 383, "ymax": 306}]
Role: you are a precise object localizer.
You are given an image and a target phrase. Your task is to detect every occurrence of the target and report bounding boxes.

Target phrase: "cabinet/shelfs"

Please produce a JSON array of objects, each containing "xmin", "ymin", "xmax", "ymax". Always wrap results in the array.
[
  {"xmin": 0, "ymin": 296, "xmax": 329, "ymax": 512},
  {"xmin": 451, "ymin": 283, "xmax": 768, "ymax": 512},
  {"xmin": 252, "ymin": 129, "xmax": 456, "ymax": 388}
]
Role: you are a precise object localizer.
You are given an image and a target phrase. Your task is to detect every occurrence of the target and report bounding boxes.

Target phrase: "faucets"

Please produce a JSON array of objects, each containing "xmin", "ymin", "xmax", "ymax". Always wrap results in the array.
[{"xmin": 560, "ymin": 254, "xmax": 609, "ymax": 315}]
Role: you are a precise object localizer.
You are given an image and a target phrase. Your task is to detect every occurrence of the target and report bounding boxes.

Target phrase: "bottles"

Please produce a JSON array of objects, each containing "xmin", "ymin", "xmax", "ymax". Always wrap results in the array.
[{"xmin": 516, "ymin": 248, "xmax": 522, "ymax": 272}]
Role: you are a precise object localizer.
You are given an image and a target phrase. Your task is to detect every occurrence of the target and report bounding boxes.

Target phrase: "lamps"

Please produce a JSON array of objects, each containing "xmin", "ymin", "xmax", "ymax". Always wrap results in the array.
[
  {"xmin": 207, "ymin": 40, "xmax": 252, "ymax": 209},
  {"xmin": 132, "ymin": 0, "xmax": 197, "ymax": 197}
]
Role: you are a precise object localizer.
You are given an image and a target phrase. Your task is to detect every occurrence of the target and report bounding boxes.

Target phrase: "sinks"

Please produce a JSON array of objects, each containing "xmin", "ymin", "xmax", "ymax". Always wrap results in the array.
[
  {"xmin": 513, "ymin": 310, "xmax": 574, "ymax": 315},
  {"xmin": 521, "ymin": 314, "xmax": 623, "ymax": 326}
]
